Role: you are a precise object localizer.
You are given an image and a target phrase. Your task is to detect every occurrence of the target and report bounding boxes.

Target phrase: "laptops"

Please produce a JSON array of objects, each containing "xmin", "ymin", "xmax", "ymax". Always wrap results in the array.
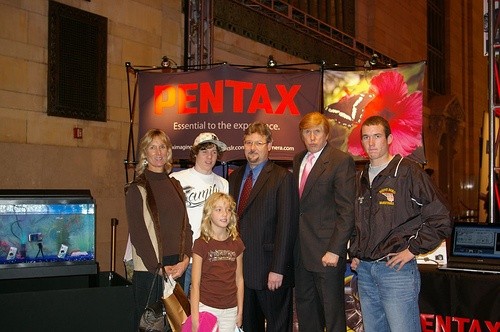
[{"xmin": 447, "ymin": 221, "xmax": 500, "ymax": 271}]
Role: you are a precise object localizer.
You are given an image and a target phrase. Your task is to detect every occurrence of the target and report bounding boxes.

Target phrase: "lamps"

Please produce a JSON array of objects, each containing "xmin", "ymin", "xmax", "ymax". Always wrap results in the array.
[
  {"xmin": 161, "ymin": 56, "xmax": 170, "ymax": 68},
  {"xmin": 267, "ymin": 53, "xmax": 276, "ymax": 67}
]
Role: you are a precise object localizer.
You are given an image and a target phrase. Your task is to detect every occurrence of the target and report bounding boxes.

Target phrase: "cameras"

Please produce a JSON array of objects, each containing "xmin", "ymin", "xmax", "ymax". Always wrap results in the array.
[
  {"xmin": 6, "ymin": 247, "xmax": 17, "ymax": 260},
  {"xmin": 28, "ymin": 233, "xmax": 43, "ymax": 241},
  {"xmin": 57, "ymin": 244, "xmax": 68, "ymax": 259}
]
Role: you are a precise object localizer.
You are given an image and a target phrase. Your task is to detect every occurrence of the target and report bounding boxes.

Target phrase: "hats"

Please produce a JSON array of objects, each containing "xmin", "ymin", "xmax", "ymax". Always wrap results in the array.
[{"xmin": 194, "ymin": 133, "xmax": 226, "ymax": 151}]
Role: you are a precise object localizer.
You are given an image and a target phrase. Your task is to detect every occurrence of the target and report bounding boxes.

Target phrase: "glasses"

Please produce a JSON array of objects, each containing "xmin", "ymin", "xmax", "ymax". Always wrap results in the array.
[{"xmin": 244, "ymin": 140, "xmax": 268, "ymax": 146}]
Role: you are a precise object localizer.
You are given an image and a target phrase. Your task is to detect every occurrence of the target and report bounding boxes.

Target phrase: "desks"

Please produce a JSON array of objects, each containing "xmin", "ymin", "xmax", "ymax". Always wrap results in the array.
[
  {"xmin": 0, "ymin": 271, "xmax": 135, "ymax": 332},
  {"xmin": 417, "ymin": 267, "xmax": 500, "ymax": 332}
]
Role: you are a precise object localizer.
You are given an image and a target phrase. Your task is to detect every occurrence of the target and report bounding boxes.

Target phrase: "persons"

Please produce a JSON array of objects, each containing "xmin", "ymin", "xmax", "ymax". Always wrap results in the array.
[
  {"xmin": 227, "ymin": 121, "xmax": 299, "ymax": 332},
  {"xmin": 293, "ymin": 112, "xmax": 356, "ymax": 332},
  {"xmin": 191, "ymin": 192, "xmax": 246, "ymax": 332},
  {"xmin": 169, "ymin": 132, "xmax": 228, "ymax": 299},
  {"xmin": 126, "ymin": 129, "xmax": 194, "ymax": 332},
  {"xmin": 348, "ymin": 116, "xmax": 453, "ymax": 332}
]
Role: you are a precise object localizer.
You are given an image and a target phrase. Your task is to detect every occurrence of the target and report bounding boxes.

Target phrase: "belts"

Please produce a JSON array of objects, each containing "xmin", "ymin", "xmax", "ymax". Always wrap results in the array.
[{"xmin": 360, "ymin": 257, "xmax": 393, "ymax": 261}]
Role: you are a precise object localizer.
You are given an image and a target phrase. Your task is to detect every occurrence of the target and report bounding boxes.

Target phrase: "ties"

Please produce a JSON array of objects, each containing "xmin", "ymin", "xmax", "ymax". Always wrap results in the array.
[
  {"xmin": 237, "ymin": 170, "xmax": 252, "ymax": 219},
  {"xmin": 299, "ymin": 154, "xmax": 315, "ymax": 199}
]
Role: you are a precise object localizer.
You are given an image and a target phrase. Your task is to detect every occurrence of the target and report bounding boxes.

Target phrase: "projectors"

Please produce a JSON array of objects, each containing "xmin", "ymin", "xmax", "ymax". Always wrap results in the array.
[{"xmin": 416, "ymin": 239, "xmax": 447, "ymax": 265}]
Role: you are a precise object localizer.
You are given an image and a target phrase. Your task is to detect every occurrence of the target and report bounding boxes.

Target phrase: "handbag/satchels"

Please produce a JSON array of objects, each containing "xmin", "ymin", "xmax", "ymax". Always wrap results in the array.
[
  {"xmin": 138, "ymin": 305, "xmax": 173, "ymax": 332},
  {"xmin": 161, "ymin": 266, "xmax": 191, "ymax": 332}
]
